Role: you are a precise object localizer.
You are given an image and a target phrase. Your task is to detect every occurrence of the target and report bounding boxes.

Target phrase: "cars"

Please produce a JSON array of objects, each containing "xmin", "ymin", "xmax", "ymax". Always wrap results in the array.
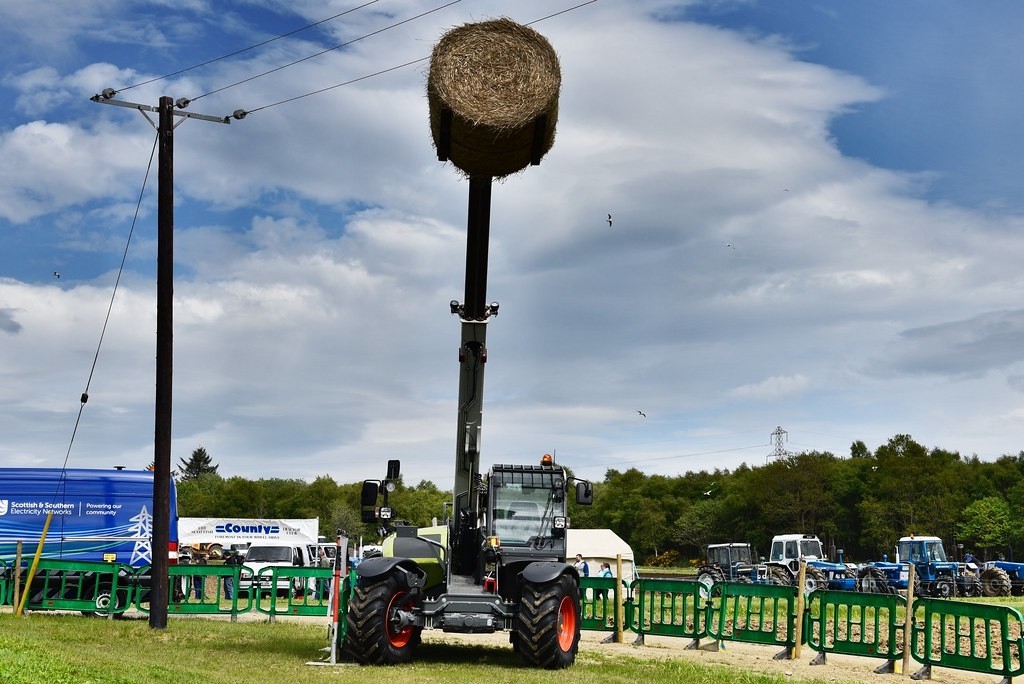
[{"xmin": 176, "ymin": 537, "xmax": 384, "ymax": 600}]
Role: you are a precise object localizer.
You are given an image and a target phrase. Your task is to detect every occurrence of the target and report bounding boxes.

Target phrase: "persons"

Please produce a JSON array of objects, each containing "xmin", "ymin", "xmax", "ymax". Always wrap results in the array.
[
  {"xmin": 181, "ymin": 555, "xmax": 191, "ymax": 566},
  {"xmin": 193, "ymin": 557, "xmax": 202, "ymax": 600},
  {"xmin": 912, "ymin": 548, "xmax": 918, "ymax": 561},
  {"xmin": 199, "ymin": 553, "xmax": 210, "ymax": 600},
  {"xmin": 221, "ymin": 551, "xmax": 236, "ymax": 600},
  {"xmin": 573, "ymin": 554, "xmax": 589, "ymax": 600},
  {"xmin": 786, "ymin": 548, "xmax": 794, "ymax": 558},
  {"xmin": 314, "ymin": 550, "xmax": 330, "ymax": 600},
  {"xmin": 239, "ymin": 556, "xmax": 245, "ymax": 565},
  {"xmin": 182, "ymin": 544, "xmax": 192, "ymax": 599},
  {"xmin": 595, "ymin": 562, "xmax": 613, "ymax": 601}
]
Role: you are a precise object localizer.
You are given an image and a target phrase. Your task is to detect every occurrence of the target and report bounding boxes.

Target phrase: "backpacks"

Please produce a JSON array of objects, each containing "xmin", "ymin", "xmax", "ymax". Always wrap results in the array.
[{"xmin": 581, "ymin": 561, "xmax": 589, "ymax": 577}]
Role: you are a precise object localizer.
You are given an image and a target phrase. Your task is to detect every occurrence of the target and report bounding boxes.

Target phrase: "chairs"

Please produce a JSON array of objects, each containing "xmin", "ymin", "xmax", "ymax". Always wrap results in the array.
[
  {"xmin": 506, "ymin": 501, "xmax": 540, "ymax": 522},
  {"xmin": 786, "ymin": 548, "xmax": 797, "ymax": 558}
]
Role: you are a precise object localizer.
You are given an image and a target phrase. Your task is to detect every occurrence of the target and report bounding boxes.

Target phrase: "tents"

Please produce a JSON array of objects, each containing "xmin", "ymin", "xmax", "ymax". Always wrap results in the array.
[{"xmin": 566, "ymin": 529, "xmax": 636, "ymax": 601}]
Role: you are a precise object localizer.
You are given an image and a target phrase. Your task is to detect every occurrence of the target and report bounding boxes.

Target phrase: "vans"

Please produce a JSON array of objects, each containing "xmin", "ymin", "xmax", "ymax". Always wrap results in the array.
[{"xmin": 0, "ymin": 463, "xmax": 182, "ymax": 620}]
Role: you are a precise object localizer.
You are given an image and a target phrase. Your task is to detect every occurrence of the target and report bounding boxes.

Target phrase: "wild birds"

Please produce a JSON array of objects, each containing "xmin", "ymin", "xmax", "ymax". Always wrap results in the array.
[
  {"xmin": 727, "ymin": 243, "xmax": 735, "ymax": 249},
  {"xmin": 637, "ymin": 411, "xmax": 646, "ymax": 418},
  {"xmin": 53, "ymin": 272, "xmax": 60, "ymax": 279},
  {"xmin": 606, "ymin": 213, "xmax": 615, "ymax": 227}
]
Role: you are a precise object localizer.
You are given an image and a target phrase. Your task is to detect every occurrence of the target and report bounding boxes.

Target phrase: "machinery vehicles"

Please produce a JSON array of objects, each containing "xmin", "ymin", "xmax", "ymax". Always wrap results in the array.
[
  {"xmin": 335, "ymin": 176, "xmax": 597, "ymax": 671},
  {"xmin": 858, "ymin": 533, "xmax": 1024, "ymax": 599},
  {"xmin": 760, "ymin": 533, "xmax": 861, "ymax": 600},
  {"xmin": 693, "ymin": 540, "xmax": 786, "ymax": 598}
]
